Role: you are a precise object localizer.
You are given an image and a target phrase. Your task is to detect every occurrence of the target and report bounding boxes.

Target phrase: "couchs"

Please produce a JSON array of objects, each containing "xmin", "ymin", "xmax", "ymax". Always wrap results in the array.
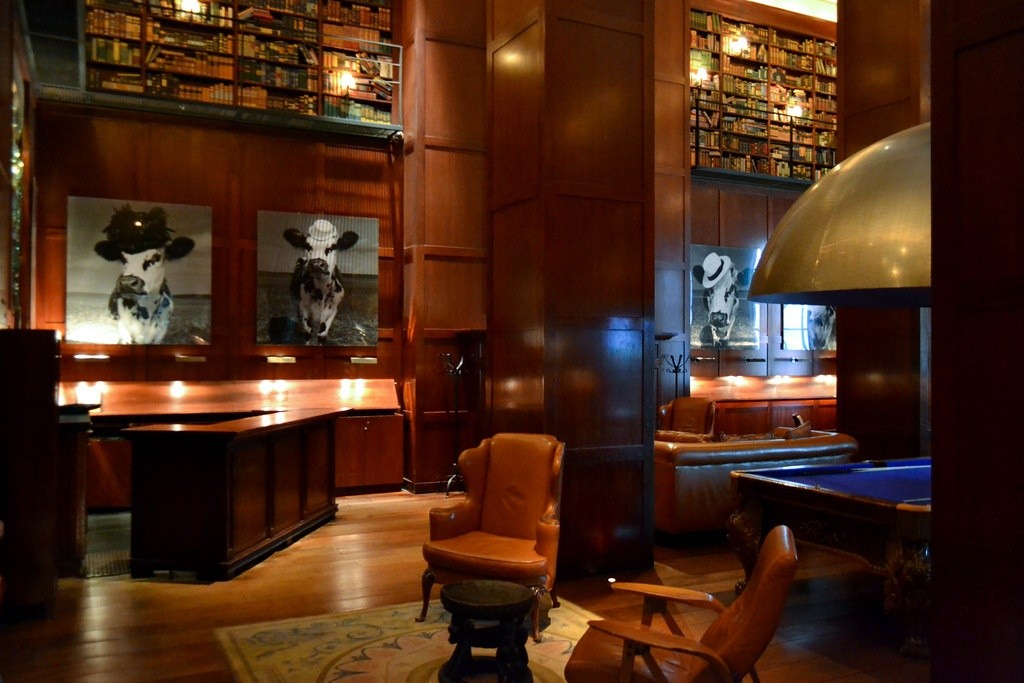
[{"xmin": 654, "ymin": 427, "xmax": 859, "ymax": 546}]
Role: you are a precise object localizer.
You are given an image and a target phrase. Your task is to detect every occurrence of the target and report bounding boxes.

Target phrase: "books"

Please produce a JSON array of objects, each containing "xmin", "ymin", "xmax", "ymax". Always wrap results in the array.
[
  {"xmin": 85, "ymin": 0, "xmax": 393, "ymax": 125},
  {"xmin": 690, "ymin": 12, "xmax": 838, "ymax": 181}
]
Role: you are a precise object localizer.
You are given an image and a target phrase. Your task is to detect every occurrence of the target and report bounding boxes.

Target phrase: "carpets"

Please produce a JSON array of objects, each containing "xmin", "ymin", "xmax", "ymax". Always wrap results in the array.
[
  {"xmin": 212, "ymin": 595, "xmax": 606, "ymax": 683},
  {"xmin": 84, "ymin": 510, "xmax": 132, "ymax": 579}
]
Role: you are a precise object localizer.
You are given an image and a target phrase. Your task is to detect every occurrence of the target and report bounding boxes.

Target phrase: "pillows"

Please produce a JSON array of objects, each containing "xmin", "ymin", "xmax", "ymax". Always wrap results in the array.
[{"xmin": 784, "ymin": 420, "xmax": 811, "ymax": 439}]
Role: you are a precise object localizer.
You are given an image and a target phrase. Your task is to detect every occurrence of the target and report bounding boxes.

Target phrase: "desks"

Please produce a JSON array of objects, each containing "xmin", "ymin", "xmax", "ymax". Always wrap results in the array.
[
  {"xmin": 440, "ymin": 580, "xmax": 534, "ymax": 683},
  {"xmin": 121, "ymin": 407, "xmax": 355, "ymax": 582},
  {"xmin": 729, "ymin": 456, "xmax": 931, "ymax": 660}
]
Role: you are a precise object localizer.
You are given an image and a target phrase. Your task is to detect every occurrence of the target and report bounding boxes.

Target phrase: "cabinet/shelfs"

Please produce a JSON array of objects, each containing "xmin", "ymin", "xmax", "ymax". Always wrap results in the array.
[
  {"xmin": 85, "ymin": 0, "xmax": 399, "ymax": 125},
  {"xmin": 691, "ymin": 7, "xmax": 836, "ymax": 182}
]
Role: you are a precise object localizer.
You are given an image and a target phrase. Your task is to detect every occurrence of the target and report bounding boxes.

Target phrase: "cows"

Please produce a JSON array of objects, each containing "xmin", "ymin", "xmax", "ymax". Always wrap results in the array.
[
  {"xmin": 94, "ymin": 205, "xmax": 197, "ymax": 344},
  {"xmin": 283, "ymin": 218, "xmax": 361, "ymax": 347},
  {"xmin": 691, "ymin": 252, "xmax": 837, "ymax": 353}
]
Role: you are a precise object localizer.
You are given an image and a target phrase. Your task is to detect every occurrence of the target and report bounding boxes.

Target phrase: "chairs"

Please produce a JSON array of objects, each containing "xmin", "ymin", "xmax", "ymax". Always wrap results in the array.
[
  {"xmin": 655, "ymin": 397, "xmax": 719, "ymax": 443},
  {"xmin": 564, "ymin": 524, "xmax": 798, "ymax": 683},
  {"xmin": 415, "ymin": 432, "xmax": 565, "ymax": 643}
]
